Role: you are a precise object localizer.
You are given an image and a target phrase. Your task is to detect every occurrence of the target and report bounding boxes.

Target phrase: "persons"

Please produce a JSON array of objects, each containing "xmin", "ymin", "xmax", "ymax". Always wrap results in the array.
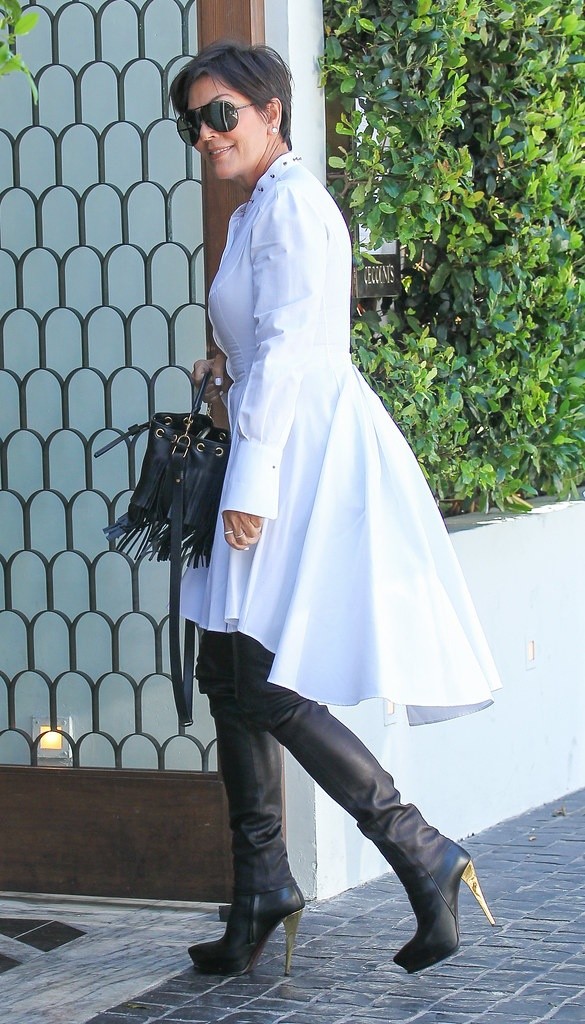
[{"xmin": 170, "ymin": 41, "xmax": 503, "ymax": 975}]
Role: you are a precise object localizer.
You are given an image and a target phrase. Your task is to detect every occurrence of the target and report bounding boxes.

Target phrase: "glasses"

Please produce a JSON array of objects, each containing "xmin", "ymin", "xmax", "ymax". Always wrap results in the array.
[{"xmin": 177, "ymin": 101, "xmax": 257, "ymax": 146}]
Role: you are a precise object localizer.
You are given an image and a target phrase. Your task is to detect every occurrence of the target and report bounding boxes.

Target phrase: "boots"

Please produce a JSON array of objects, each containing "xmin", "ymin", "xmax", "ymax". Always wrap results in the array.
[
  {"xmin": 270, "ymin": 697, "xmax": 496, "ymax": 971},
  {"xmin": 187, "ymin": 701, "xmax": 305, "ymax": 976}
]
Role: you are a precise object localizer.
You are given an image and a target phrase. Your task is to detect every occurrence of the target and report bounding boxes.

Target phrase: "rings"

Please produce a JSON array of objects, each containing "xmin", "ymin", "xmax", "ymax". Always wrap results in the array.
[
  {"xmin": 225, "ymin": 530, "xmax": 233, "ymax": 535},
  {"xmin": 235, "ymin": 533, "xmax": 245, "ymax": 538}
]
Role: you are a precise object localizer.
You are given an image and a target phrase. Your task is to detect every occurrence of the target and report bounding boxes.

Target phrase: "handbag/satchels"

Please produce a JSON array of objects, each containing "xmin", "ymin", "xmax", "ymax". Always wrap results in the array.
[{"xmin": 94, "ymin": 370, "xmax": 232, "ymax": 569}]
